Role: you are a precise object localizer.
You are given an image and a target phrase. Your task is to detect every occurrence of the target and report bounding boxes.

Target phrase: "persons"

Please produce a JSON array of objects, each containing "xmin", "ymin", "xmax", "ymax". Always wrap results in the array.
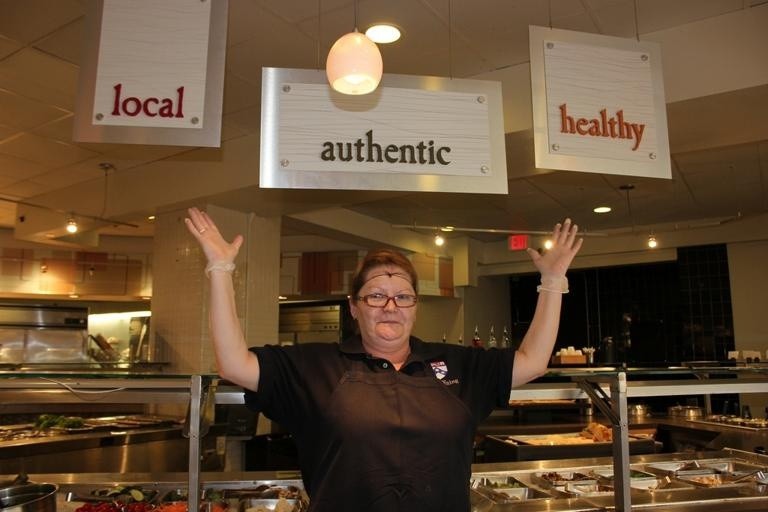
[{"xmin": 183, "ymin": 204, "xmax": 584, "ymax": 512}]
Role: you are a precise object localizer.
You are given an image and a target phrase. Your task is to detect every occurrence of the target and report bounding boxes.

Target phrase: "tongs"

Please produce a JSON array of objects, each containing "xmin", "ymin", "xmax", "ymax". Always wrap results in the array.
[
  {"xmin": 222, "ymin": 485, "xmax": 278, "ymax": 499},
  {"xmin": 472, "ymin": 480, "xmax": 508, "ymax": 504},
  {"xmin": 656, "ymin": 476, "xmax": 671, "ymax": 489},
  {"xmin": 565, "ymin": 482, "xmax": 585, "ymax": 496},
  {"xmin": 723, "ymin": 471, "xmax": 765, "ymax": 485},
  {"xmin": 66, "ymin": 491, "xmax": 131, "ymax": 506}
]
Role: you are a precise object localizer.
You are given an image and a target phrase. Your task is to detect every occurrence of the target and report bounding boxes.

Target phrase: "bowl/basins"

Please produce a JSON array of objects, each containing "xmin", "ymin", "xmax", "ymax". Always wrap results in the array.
[{"xmin": 1, "ymin": 480, "xmax": 60, "ymax": 512}]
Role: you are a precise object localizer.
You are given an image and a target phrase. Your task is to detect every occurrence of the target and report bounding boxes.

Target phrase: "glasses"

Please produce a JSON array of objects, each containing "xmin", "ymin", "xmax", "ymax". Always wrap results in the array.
[{"xmin": 354, "ymin": 293, "xmax": 417, "ymax": 308}]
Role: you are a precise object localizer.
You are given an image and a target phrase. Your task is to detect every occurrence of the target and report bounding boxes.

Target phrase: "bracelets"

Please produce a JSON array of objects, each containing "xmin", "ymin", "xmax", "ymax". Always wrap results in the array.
[
  {"xmin": 202, "ymin": 261, "xmax": 237, "ymax": 277},
  {"xmin": 536, "ymin": 277, "xmax": 570, "ymax": 295}
]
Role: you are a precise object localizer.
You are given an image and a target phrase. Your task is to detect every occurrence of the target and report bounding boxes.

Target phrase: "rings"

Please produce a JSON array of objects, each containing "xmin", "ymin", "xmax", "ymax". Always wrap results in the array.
[{"xmin": 199, "ymin": 228, "xmax": 205, "ymax": 233}]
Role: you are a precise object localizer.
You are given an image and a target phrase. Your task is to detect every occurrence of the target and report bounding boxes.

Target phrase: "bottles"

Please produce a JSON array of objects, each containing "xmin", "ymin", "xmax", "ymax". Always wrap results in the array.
[{"xmin": 441, "ymin": 325, "xmax": 512, "ymax": 347}]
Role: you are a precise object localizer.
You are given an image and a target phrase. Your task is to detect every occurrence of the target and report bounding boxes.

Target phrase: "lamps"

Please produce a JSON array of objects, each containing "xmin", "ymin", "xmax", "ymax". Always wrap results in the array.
[
  {"xmin": 392, "ymin": 186, "xmax": 609, "ymax": 250},
  {"xmin": 0, "ymin": 162, "xmax": 140, "ymax": 235},
  {"xmin": 326, "ymin": 0, "xmax": 384, "ymax": 95},
  {"xmin": 610, "ymin": 185, "xmax": 745, "ymax": 249}
]
